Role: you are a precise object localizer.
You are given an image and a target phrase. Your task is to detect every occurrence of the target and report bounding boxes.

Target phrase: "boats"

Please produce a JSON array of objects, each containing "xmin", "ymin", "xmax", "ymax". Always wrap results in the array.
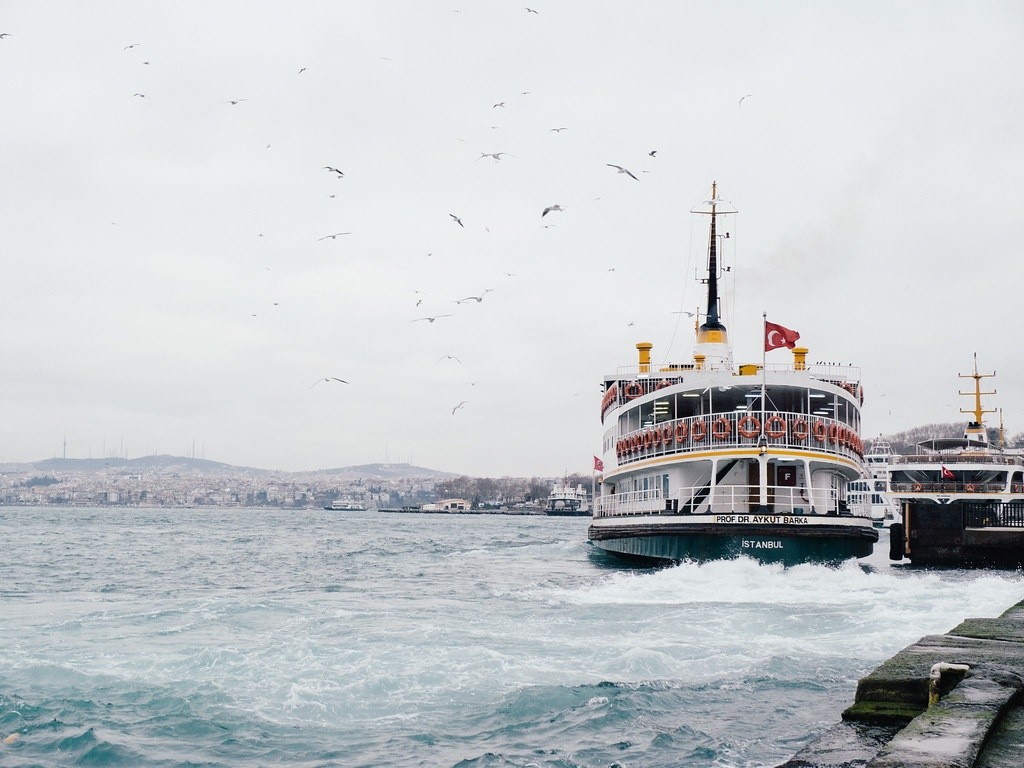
[
  {"xmin": 542, "ymin": 468, "xmax": 589, "ymax": 516},
  {"xmin": 888, "ymin": 352, "xmax": 1024, "ymax": 569},
  {"xmin": 587, "ymin": 179, "xmax": 880, "ymax": 568},
  {"xmin": 847, "ymin": 431, "xmax": 902, "ymax": 528},
  {"xmin": 323, "ymin": 499, "xmax": 369, "ymax": 511}
]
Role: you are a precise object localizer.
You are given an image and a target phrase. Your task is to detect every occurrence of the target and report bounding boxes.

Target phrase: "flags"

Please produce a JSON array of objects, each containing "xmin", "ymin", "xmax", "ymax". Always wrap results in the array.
[
  {"xmin": 765, "ymin": 321, "xmax": 800, "ymax": 352},
  {"xmin": 593, "ymin": 454, "xmax": 603, "ymax": 471},
  {"xmin": 941, "ymin": 465, "xmax": 955, "ymax": 479}
]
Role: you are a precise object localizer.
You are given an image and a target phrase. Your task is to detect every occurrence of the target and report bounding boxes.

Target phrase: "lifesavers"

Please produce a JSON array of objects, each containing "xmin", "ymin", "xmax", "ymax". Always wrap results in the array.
[
  {"xmin": 615, "ymin": 418, "xmax": 707, "ymax": 459},
  {"xmin": 598, "ymin": 382, "xmax": 619, "ymax": 427},
  {"xmin": 655, "ymin": 380, "xmax": 674, "ymax": 392},
  {"xmin": 965, "ymin": 482, "xmax": 975, "ymax": 493},
  {"xmin": 840, "ymin": 383, "xmax": 854, "ymax": 397},
  {"xmin": 912, "ymin": 481, "xmax": 923, "ymax": 492},
  {"xmin": 1013, "ymin": 483, "xmax": 1020, "ymax": 495},
  {"xmin": 736, "ymin": 415, "xmax": 762, "ymax": 439},
  {"xmin": 763, "ymin": 414, "xmax": 788, "ymax": 440},
  {"xmin": 859, "ymin": 384, "xmax": 866, "ymax": 405},
  {"xmin": 623, "ymin": 381, "xmax": 644, "ymax": 401},
  {"xmin": 791, "ymin": 417, "xmax": 865, "ymax": 458},
  {"xmin": 711, "ymin": 416, "xmax": 732, "ymax": 441}
]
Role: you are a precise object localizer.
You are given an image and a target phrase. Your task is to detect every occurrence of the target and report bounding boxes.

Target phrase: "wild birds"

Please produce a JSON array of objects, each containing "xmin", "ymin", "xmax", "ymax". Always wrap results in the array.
[{"xmin": 0, "ymin": 6, "xmax": 757, "ymax": 420}]
